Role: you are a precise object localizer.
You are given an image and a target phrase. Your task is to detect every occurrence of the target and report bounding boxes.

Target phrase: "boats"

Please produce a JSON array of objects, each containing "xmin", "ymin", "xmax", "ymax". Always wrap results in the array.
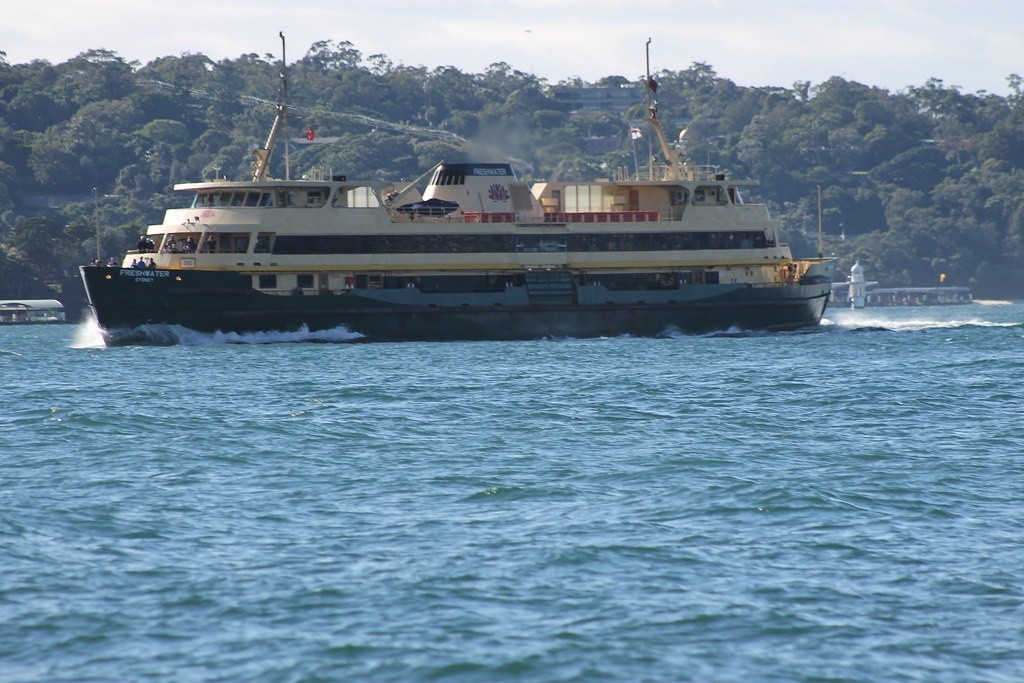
[{"xmin": 79, "ymin": 30, "xmax": 839, "ymax": 352}]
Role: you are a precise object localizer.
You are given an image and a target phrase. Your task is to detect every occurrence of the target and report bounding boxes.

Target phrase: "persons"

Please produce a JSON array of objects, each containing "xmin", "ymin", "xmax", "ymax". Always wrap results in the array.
[
  {"xmin": 320, "ymin": 281, "xmax": 328, "ymax": 295},
  {"xmin": 90, "ymin": 234, "xmax": 216, "ymax": 269},
  {"xmin": 292, "ymin": 282, "xmax": 304, "ymax": 296}
]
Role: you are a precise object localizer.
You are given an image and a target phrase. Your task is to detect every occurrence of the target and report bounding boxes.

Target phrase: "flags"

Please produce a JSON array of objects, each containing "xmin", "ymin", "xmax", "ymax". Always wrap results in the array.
[
  {"xmin": 649, "ymin": 77, "xmax": 657, "ymax": 94},
  {"xmin": 631, "ymin": 126, "xmax": 642, "ymax": 139}
]
